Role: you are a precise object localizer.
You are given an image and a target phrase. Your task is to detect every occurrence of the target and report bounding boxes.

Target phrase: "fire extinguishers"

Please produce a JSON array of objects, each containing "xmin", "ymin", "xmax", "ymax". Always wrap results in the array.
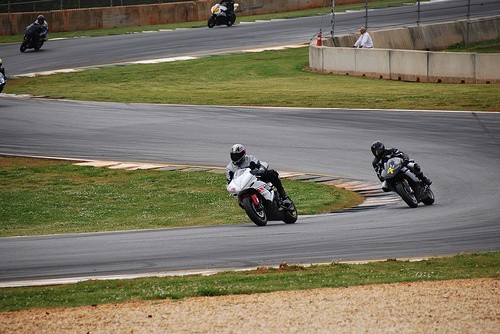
[{"xmin": 317, "ymin": 32, "xmax": 322, "ymax": 46}]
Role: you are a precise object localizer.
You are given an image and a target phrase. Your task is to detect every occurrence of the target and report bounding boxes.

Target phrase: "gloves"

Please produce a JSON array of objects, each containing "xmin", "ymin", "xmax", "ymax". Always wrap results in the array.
[{"xmin": 256, "ymin": 171, "xmax": 264, "ymax": 178}]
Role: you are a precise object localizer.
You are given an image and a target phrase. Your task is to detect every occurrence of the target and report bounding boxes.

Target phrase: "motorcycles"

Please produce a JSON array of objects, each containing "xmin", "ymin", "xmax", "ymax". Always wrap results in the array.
[
  {"xmin": 225, "ymin": 167, "xmax": 298, "ymax": 227},
  {"xmin": 0, "ymin": 59, "xmax": 7, "ymax": 93},
  {"xmin": 20, "ymin": 22, "xmax": 47, "ymax": 52},
  {"xmin": 207, "ymin": 3, "xmax": 239, "ymax": 28},
  {"xmin": 381, "ymin": 156, "xmax": 435, "ymax": 208}
]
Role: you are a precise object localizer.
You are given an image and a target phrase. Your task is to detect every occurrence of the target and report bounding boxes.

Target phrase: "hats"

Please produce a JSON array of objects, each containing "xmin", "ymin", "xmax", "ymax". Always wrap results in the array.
[{"xmin": 359, "ymin": 27, "xmax": 366, "ymax": 32}]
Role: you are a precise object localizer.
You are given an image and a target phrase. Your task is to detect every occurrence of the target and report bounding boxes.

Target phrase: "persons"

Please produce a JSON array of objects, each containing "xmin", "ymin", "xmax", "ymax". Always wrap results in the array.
[
  {"xmin": 219, "ymin": 0, "xmax": 234, "ymax": 14},
  {"xmin": 353, "ymin": 26, "xmax": 373, "ymax": 48},
  {"xmin": 26, "ymin": 14, "xmax": 49, "ymax": 38},
  {"xmin": 0, "ymin": 59, "xmax": 6, "ymax": 80},
  {"xmin": 371, "ymin": 140, "xmax": 432, "ymax": 192},
  {"xmin": 226, "ymin": 144, "xmax": 291, "ymax": 206}
]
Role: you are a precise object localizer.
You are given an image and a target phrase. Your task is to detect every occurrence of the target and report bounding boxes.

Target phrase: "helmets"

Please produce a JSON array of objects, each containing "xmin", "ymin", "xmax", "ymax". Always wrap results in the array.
[
  {"xmin": 229, "ymin": 143, "xmax": 246, "ymax": 164},
  {"xmin": 370, "ymin": 142, "xmax": 384, "ymax": 157},
  {"xmin": 37, "ymin": 15, "xmax": 45, "ymax": 24}
]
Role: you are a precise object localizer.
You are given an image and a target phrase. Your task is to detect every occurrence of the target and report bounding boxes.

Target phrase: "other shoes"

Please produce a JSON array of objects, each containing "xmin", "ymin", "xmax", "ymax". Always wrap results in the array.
[
  {"xmin": 279, "ymin": 190, "xmax": 286, "ymax": 196},
  {"xmin": 426, "ymin": 179, "xmax": 433, "ymax": 185}
]
